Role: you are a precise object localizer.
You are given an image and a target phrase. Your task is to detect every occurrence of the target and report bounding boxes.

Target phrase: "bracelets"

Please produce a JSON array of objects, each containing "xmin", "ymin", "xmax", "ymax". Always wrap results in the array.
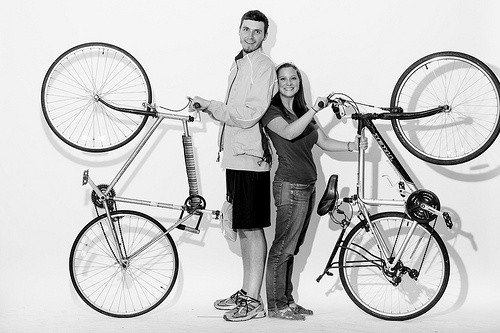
[
  {"xmin": 311, "ymin": 107, "xmax": 317, "ymax": 113},
  {"xmin": 347, "ymin": 142, "xmax": 353, "ymax": 152}
]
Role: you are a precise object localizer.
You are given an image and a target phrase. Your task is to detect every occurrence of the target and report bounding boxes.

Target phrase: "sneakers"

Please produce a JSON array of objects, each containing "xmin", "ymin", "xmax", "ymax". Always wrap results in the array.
[
  {"xmin": 213, "ymin": 289, "xmax": 245, "ymax": 310},
  {"xmin": 293, "ymin": 301, "xmax": 315, "ymax": 314},
  {"xmin": 270, "ymin": 304, "xmax": 306, "ymax": 322},
  {"xmin": 222, "ymin": 297, "xmax": 265, "ymax": 324}
]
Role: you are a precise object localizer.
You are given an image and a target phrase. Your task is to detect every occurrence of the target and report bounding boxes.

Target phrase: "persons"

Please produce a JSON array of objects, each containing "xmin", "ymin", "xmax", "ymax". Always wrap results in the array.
[
  {"xmin": 188, "ymin": 10, "xmax": 279, "ymax": 321},
  {"xmin": 261, "ymin": 63, "xmax": 368, "ymax": 320}
]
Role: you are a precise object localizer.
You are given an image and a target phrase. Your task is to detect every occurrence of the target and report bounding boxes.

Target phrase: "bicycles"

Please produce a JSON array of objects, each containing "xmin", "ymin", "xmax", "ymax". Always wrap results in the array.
[
  {"xmin": 316, "ymin": 50, "xmax": 500, "ymax": 322},
  {"xmin": 40, "ymin": 41, "xmax": 237, "ymax": 320}
]
